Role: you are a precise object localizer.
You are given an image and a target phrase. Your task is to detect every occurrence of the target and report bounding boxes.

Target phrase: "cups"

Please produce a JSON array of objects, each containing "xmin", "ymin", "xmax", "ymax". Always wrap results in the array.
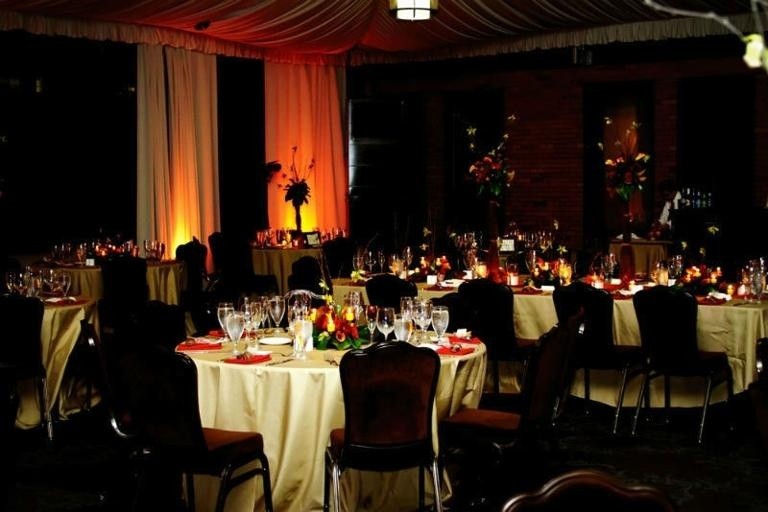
[
  {"xmin": 413, "ymin": 297, "xmax": 426, "ymax": 333},
  {"xmin": 244, "ymin": 297, "xmax": 268, "ymax": 338},
  {"xmin": 400, "ymin": 297, "xmax": 410, "ymax": 319},
  {"xmin": 240, "ymin": 305, "xmax": 252, "ymax": 344},
  {"xmin": 227, "ymin": 311, "xmax": 244, "ymax": 355},
  {"xmin": 418, "ymin": 301, "xmax": 432, "ymax": 340},
  {"xmin": 218, "ymin": 304, "xmax": 232, "ymax": 344},
  {"xmin": 252, "ymin": 303, "xmax": 260, "ymax": 351},
  {"xmin": 430, "ymin": 306, "xmax": 448, "ymax": 344},
  {"xmin": 394, "ymin": 313, "xmax": 412, "ymax": 341},
  {"xmin": 269, "ymin": 296, "xmax": 285, "ymax": 331},
  {"xmin": 377, "ymin": 308, "xmax": 394, "ymax": 344},
  {"xmin": 365, "ymin": 306, "xmax": 377, "ymax": 346}
]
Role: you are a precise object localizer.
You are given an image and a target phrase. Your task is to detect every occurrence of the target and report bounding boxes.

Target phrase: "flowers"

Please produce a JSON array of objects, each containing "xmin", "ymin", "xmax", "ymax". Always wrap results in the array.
[
  {"xmin": 252, "ymin": 144, "xmax": 315, "ymax": 204},
  {"xmin": 597, "ymin": 116, "xmax": 650, "ymax": 204},
  {"xmin": 464, "ymin": 115, "xmax": 517, "ymax": 205}
]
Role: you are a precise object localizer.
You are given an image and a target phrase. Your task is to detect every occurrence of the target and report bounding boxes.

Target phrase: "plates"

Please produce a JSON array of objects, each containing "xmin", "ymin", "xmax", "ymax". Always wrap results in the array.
[{"xmin": 258, "ymin": 337, "xmax": 292, "ymax": 344}]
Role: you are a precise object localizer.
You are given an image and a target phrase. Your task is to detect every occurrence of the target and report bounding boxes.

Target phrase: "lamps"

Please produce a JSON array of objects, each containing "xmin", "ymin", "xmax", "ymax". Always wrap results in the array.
[
  {"xmin": 741, "ymin": 0, "xmax": 766, "ymax": 69},
  {"xmin": 389, "ymin": 0, "xmax": 439, "ymax": 22}
]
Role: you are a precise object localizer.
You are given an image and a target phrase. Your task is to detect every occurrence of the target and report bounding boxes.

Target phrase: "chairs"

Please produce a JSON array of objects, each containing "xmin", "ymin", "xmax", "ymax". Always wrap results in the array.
[
  {"xmin": 365, "ymin": 274, "xmax": 417, "ymax": 324},
  {"xmin": 547, "ymin": 282, "xmax": 649, "ymax": 435},
  {"xmin": 242, "ymin": 274, "xmax": 285, "ymax": 328},
  {"xmin": 105, "ymin": 300, "xmax": 186, "ymax": 512},
  {"xmin": 322, "ymin": 237, "xmax": 357, "ymax": 295},
  {"xmin": 630, "ymin": 285, "xmax": 734, "ymax": 445},
  {"xmin": 113, "ymin": 346, "xmax": 272, "ymax": 512},
  {"xmin": 325, "ymin": 342, "xmax": 440, "ymax": 512},
  {"xmin": 177, "ymin": 241, "xmax": 208, "ymax": 330},
  {"xmin": 287, "ymin": 255, "xmax": 327, "ymax": 308},
  {"xmin": 0, "ymin": 294, "xmax": 55, "ymax": 450},
  {"xmin": 208, "ymin": 231, "xmax": 278, "ymax": 311},
  {"xmin": 363, "ymin": 230, "xmax": 404, "ymax": 274},
  {"xmin": 204, "ymin": 274, "xmax": 247, "ymax": 334},
  {"xmin": 501, "ymin": 470, "xmax": 676, "ymax": 512},
  {"xmin": 438, "ymin": 334, "xmax": 555, "ymax": 497},
  {"xmin": 456, "ymin": 280, "xmax": 540, "ymax": 409},
  {"xmin": 67, "ymin": 252, "xmax": 150, "ymax": 413}
]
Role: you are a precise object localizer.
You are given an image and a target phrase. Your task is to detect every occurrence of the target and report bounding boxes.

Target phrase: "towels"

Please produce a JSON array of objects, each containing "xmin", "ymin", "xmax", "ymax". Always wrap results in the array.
[
  {"xmin": 437, "ymin": 346, "xmax": 474, "ymax": 355},
  {"xmin": 449, "ymin": 335, "xmax": 480, "ymax": 344},
  {"xmin": 224, "ymin": 353, "xmax": 271, "ymax": 364},
  {"xmin": 209, "ymin": 330, "xmax": 255, "ymax": 337},
  {"xmin": 177, "ymin": 342, "xmax": 222, "ymax": 351}
]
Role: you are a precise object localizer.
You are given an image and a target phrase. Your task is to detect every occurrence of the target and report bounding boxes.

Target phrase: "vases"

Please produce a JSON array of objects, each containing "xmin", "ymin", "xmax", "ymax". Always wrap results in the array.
[
  {"xmin": 495, "ymin": 200, "xmax": 507, "ymax": 238},
  {"xmin": 622, "ymin": 203, "xmax": 633, "ymax": 243}
]
